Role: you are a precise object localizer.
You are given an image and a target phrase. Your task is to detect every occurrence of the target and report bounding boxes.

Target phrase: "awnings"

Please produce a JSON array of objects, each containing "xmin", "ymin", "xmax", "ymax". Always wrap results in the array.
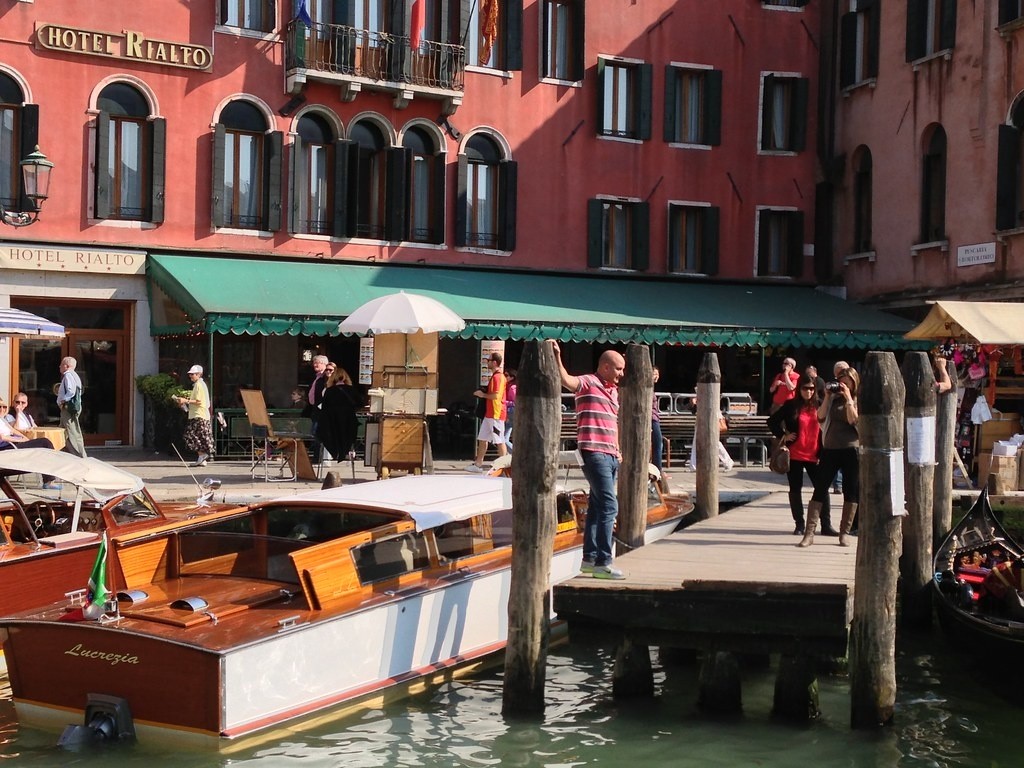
[{"xmin": 143, "ymin": 253, "xmax": 944, "ymax": 349}]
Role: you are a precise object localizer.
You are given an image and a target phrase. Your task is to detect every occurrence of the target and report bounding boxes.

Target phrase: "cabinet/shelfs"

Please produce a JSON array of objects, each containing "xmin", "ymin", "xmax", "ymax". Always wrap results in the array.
[{"xmin": 377, "ymin": 415, "xmax": 428, "ymax": 480}]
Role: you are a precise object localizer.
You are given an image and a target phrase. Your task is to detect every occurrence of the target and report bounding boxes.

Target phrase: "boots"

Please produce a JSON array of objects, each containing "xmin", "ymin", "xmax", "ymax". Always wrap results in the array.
[
  {"xmin": 818, "ymin": 494, "xmax": 840, "ymax": 536},
  {"xmin": 798, "ymin": 500, "xmax": 823, "ymax": 547},
  {"xmin": 788, "ymin": 492, "xmax": 805, "ymax": 535},
  {"xmin": 839, "ymin": 501, "xmax": 858, "ymax": 547}
]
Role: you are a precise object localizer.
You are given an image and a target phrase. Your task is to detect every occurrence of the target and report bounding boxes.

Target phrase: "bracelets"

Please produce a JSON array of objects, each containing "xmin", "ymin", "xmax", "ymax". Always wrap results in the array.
[
  {"xmin": 187, "ymin": 400, "xmax": 190, "ymax": 404},
  {"xmin": 845, "ymin": 400, "xmax": 854, "ymax": 406}
]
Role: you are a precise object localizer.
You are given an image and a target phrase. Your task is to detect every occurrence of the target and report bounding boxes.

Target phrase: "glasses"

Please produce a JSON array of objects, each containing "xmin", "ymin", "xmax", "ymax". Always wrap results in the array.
[
  {"xmin": 783, "ymin": 362, "xmax": 788, "ymax": 365},
  {"xmin": 0, "ymin": 405, "xmax": 7, "ymax": 409},
  {"xmin": 324, "ymin": 368, "xmax": 333, "ymax": 372},
  {"xmin": 486, "ymin": 358, "xmax": 494, "ymax": 362},
  {"xmin": 14, "ymin": 400, "xmax": 26, "ymax": 404},
  {"xmin": 800, "ymin": 386, "xmax": 815, "ymax": 391},
  {"xmin": 290, "ymin": 392, "xmax": 295, "ymax": 395},
  {"xmin": 504, "ymin": 373, "xmax": 511, "ymax": 377}
]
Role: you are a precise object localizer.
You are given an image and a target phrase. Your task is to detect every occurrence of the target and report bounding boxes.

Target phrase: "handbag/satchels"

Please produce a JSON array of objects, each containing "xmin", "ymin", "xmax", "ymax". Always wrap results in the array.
[
  {"xmin": 717, "ymin": 415, "xmax": 731, "ymax": 432},
  {"xmin": 66, "ymin": 385, "xmax": 80, "ymax": 413},
  {"xmin": 767, "ymin": 434, "xmax": 791, "ymax": 475},
  {"xmin": 473, "ymin": 386, "xmax": 488, "ymax": 420}
]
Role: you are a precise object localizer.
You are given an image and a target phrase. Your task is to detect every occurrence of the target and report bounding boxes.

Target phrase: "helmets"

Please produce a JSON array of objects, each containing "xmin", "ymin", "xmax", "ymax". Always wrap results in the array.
[{"xmin": 647, "ymin": 462, "xmax": 662, "ymax": 482}]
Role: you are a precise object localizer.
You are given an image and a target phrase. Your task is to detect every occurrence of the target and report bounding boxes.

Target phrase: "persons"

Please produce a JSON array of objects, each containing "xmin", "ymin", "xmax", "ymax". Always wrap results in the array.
[
  {"xmin": 0, "ymin": 396, "xmax": 64, "ymax": 490},
  {"xmin": 174, "ymin": 363, "xmax": 214, "ymax": 467},
  {"xmin": 765, "ymin": 357, "xmax": 862, "ymax": 547},
  {"xmin": 501, "ymin": 367, "xmax": 518, "ymax": 454},
  {"xmin": 544, "ymin": 337, "xmax": 627, "ymax": 580},
  {"xmin": 4, "ymin": 393, "xmax": 38, "ymax": 431},
  {"xmin": 927, "ymin": 353, "xmax": 951, "ymax": 394},
  {"xmin": 684, "ymin": 386, "xmax": 735, "ymax": 474},
  {"xmin": 55, "ymin": 357, "xmax": 88, "ymax": 459},
  {"xmin": 463, "ymin": 352, "xmax": 506, "ymax": 473},
  {"xmin": 290, "ymin": 355, "xmax": 358, "ymax": 466},
  {"xmin": 650, "ymin": 366, "xmax": 674, "ymax": 480}
]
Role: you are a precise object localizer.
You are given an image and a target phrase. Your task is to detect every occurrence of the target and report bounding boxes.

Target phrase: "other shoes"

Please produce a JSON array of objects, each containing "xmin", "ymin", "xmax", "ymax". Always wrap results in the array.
[
  {"xmin": 189, "ymin": 454, "xmax": 209, "ymax": 467},
  {"xmin": 43, "ymin": 483, "xmax": 63, "ymax": 490}
]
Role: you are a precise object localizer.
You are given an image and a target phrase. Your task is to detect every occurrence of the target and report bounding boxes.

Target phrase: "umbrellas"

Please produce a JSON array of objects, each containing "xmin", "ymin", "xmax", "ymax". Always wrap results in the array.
[
  {"xmin": 0, "ymin": 307, "xmax": 68, "ymax": 342},
  {"xmin": 336, "ymin": 288, "xmax": 468, "ymax": 383}
]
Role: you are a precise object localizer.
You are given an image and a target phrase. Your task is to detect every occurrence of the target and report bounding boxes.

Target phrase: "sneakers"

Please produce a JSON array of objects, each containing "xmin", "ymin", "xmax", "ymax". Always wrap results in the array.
[
  {"xmin": 592, "ymin": 567, "xmax": 626, "ymax": 580},
  {"xmin": 462, "ymin": 464, "xmax": 483, "ymax": 472},
  {"xmin": 580, "ymin": 559, "xmax": 611, "ymax": 575}
]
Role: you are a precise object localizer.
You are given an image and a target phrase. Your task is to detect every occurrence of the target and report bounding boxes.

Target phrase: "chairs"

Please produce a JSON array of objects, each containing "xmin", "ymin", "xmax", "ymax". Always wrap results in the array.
[
  {"xmin": 6, "ymin": 441, "xmax": 41, "ymax": 490},
  {"xmin": 251, "ymin": 422, "xmax": 300, "ymax": 482}
]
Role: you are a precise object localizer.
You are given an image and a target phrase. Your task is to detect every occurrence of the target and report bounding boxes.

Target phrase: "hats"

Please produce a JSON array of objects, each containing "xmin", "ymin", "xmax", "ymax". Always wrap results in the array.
[{"xmin": 187, "ymin": 365, "xmax": 204, "ymax": 374}]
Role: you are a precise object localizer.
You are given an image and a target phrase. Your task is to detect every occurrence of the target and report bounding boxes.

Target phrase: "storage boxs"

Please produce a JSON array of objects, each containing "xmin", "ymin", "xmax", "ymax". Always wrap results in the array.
[{"xmin": 977, "ymin": 441, "xmax": 1024, "ymax": 494}]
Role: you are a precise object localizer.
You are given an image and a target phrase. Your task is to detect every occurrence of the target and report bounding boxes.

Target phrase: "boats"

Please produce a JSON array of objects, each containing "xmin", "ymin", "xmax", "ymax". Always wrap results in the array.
[
  {"xmin": 931, "ymin": 473, "xmax": 1024, "ymax": 703},
  {"xmin": 0, "ymin": 448, "xmax": 250, "ymax": 703},
  {"xmin": 0, "ymin": 445, "xmax": 699, "ymax": 757}
]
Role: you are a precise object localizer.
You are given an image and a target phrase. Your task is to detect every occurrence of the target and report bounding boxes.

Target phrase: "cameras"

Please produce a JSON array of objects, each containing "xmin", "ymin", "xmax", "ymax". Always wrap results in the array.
[{"xmin": 826, "ymin": 381, "xmax": 841, "ymax": 393}]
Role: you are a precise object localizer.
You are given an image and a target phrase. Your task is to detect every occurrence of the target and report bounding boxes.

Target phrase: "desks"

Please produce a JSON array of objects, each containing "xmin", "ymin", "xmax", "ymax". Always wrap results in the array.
[{"xmin": 18, "ymin": 427, "xmax": 65, "ymax": 487}]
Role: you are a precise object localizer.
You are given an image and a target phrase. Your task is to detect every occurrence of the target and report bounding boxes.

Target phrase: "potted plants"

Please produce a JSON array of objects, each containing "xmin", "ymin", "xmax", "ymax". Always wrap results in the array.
[{"xmin": 134, "ymin": 373, "xmax": 193, "ymax": 457}]
